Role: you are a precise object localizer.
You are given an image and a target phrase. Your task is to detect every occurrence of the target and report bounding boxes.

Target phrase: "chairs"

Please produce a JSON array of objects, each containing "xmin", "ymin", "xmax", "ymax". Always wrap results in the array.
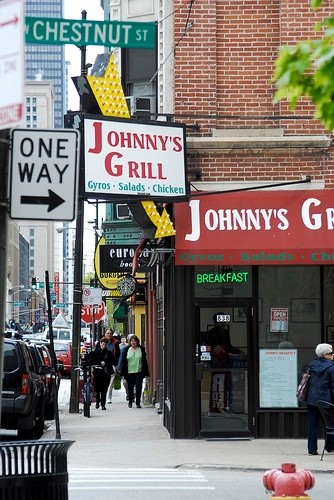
[{"xmin": 315, "ymin": 399, "xmax": 333, "ymax": 462}]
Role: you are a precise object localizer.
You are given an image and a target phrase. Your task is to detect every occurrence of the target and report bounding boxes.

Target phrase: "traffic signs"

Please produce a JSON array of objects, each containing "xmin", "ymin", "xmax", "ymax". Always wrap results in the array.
[
  {"xmin": 78, "ymin": 113, "xmax": 191, "ymax": 202},
  {"xmin": 10, "ymin": 128, "xmax": 80, "ymax": 222},
  {"xmin": 21, "ymin": 15, "xmax": 158, "ymax": 51}
]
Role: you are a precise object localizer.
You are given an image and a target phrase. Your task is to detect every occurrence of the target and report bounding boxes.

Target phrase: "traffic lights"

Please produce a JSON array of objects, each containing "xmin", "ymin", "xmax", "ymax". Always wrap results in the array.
[
  {"xmin": 90, "ymin": 278, "xmax": 94, "ymax": 287},
  {"xmin": 31, "ymin": 276, "xmax": 36, "ymax": 289},
  {"xmin": 53, "ymin": 300, "xmax": 56, "ymax": 304},
  {"xmin": 25, "ymin": 297, "xmax": 29, "ymax": 306}
]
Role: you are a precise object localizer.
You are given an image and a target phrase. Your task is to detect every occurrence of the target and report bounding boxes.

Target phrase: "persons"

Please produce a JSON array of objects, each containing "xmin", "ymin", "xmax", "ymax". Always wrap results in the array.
[
  {"xmin": 89, "ymin": 337, "xmax": 114, "ymax": 410},
  {"xmin": 118, "ymin": 336, "xmax": 129, "ymax": 351},
  {"xmin": 206, "ymin": 311, "xmax": 245, "ymax": 416},
  {"xmin": 305, "ymin": 342, "xmax": 334, "ymax": 454},
  {"xmin": 116, "ymin": 333, "xmax": 136, "ymax": 403},
  {"xmin": 96, "ymin": 328, "xmax": 120, "ymax": 404},
  {"xmin": 117, "ymin": 335, "xmax": 150, "ymax": 408}
]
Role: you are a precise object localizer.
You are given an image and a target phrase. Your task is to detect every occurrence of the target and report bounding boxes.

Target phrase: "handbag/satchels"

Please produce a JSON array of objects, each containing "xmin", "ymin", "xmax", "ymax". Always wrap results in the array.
[
  {"xmin": 113, "ymin": 372, "xmax": 121, "ymax": 390},
  {"xmin": 143, "ymin": 378, "xmax": 153, "ymax": 405},
  {"xmin": 296, "ymin": 367, "xmax": 311, "ymax": 404},
  {"xmin": 104, "ymin": 349, "xmax": 114, "ymax": 375}
]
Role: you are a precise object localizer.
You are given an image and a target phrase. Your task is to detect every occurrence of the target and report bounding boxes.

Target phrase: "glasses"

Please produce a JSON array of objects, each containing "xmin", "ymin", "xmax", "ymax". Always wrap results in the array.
[{"xmin": 327, "ymin": 352, "xmax": 334, "ymax": 355}]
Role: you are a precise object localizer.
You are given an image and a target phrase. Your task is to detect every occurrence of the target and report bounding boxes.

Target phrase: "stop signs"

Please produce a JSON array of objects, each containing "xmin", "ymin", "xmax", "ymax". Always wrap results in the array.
[{"xmin": 81, "ymin": 301, "xmax": 106, "ymax": 324}]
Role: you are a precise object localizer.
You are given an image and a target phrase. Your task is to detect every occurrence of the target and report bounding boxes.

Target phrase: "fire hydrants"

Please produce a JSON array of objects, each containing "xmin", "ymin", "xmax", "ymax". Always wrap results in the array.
[{"xmin": 262, "ymin": 462, "xmax": 316, "ymax": 500}]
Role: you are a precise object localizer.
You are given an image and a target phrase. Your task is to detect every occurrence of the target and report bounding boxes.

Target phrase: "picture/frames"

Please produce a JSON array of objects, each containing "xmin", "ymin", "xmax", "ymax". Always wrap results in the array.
[
  {"xmin": 326, "ymin": 325, "xmax": 334, "ymax": 344},
  {"xmin": 232, "ymin": 297, "xmax": 263, "ymax": 323},
  {"xmin": 291, "ymin": 297, "xmax": 322, "ymax": 323},
  {"xmin": 265, "ymin": 324, "xmax": 288, "ymax": 343}
]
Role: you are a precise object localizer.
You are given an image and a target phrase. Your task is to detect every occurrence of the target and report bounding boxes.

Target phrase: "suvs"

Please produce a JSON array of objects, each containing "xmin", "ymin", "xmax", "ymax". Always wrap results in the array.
[
  {"xmin": 22, "ymin": 338, "xmax": 65, "ymax": 420},
  {"xmin": 2, "ymin": 338, "xmax": 53, "ymax": 440},
  {"xmin": 47, "ymin": 338, "xmax": 83, "ymax": 380}
]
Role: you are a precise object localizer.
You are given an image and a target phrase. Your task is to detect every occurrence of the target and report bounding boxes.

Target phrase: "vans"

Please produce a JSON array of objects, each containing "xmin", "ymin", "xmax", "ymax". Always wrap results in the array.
[{"xmin": 44, "ymin": 328, "xmax": 72, "ymax": 340}]
